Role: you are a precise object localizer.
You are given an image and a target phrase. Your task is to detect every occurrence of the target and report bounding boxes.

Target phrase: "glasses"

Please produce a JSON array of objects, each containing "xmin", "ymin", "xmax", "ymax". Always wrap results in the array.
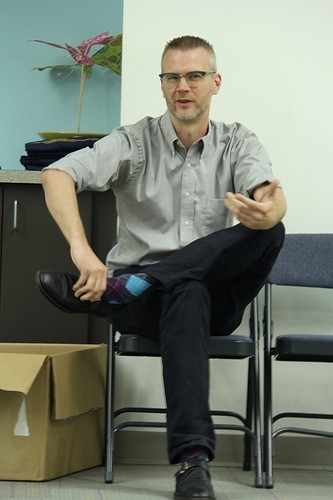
[{"xmin": 159, "ymin": 70, "xmax": 216, "ymax": 83}]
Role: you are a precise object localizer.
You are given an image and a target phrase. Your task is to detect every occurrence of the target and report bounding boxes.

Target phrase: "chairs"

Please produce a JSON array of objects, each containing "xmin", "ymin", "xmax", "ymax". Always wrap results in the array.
[
  {"xmin": 265, "ymin": 234, "xmax": 333, "ymax": 489},
  {"xmin": 104, "ymin": 295, "xmax": 262, "ymax": 487}
]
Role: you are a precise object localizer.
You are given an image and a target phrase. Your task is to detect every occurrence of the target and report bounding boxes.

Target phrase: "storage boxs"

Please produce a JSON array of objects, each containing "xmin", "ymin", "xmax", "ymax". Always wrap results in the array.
[{"xmin": 1, "ymin": 342, "xmax": 108, "ymax": 482}]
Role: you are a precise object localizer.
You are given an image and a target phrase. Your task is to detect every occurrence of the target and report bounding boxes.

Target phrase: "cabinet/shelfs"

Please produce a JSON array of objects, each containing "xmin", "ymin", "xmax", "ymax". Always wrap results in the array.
[{"xmin": 0, "ymin": 170, "xmax": 118, "ymax": 345}]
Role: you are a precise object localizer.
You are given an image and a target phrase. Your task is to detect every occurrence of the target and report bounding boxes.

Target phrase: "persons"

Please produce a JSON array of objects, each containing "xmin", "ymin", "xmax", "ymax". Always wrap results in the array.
[{"xmin": 41, "ymin": 35, "xmax": 286, "ymax": 500}]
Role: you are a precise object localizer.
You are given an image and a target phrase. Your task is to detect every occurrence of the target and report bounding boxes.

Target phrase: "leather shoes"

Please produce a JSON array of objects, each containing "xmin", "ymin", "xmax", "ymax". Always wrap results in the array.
[
  {"xmin": 33, "ymin": 268, "xmax": 129, "ymax": 318},
  {"xmin": 173, "ymin": 458, "xmax": 216, "ymax": 500}
]
